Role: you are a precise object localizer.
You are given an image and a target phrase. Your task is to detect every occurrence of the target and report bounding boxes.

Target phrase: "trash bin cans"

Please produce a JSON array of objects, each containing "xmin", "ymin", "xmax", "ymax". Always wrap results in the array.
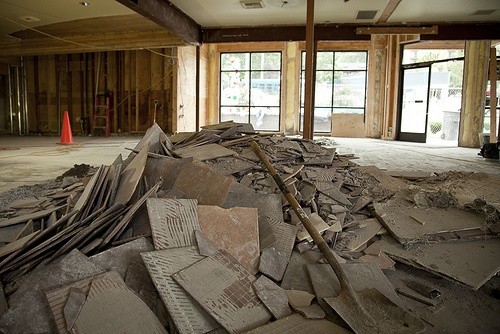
[{"xmin": 478, "ymin": 133, "xmax": 490, "ymax": 149}]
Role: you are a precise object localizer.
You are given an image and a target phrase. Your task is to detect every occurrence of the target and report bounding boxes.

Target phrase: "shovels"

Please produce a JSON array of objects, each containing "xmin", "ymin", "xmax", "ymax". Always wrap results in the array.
[{"xmin": 248, "ymin": 138, "xmax": 428, "ymax": 334}]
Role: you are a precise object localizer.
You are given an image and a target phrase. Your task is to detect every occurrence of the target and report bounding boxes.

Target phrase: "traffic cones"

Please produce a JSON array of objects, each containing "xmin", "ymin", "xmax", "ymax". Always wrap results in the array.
[{"xmin": 56, "ymin": 110, "xmax": 79, "ymax": 145}]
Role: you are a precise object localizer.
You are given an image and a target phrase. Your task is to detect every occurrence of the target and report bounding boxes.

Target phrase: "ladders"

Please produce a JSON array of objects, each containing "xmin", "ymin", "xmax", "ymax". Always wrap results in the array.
[{"xmin": 89, "ymin": 93, "xmax": 111, "ymax": 138}]
